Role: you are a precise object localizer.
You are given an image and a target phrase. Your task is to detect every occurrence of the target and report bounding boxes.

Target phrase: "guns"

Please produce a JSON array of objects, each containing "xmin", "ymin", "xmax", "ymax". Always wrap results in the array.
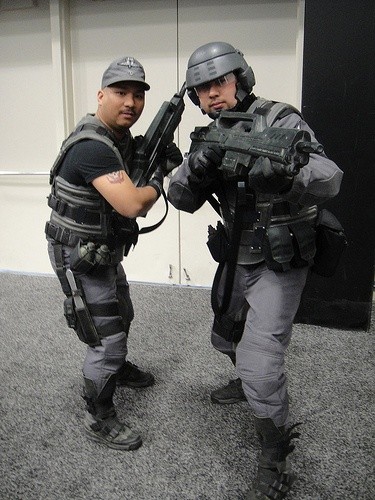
[
  {"xmin": 189, "ymin": 111, "xmax": 325, "ymax": 176},
  {"xmin": 111, "ymin": 80, "xmax": 187, "ymax": 239}
]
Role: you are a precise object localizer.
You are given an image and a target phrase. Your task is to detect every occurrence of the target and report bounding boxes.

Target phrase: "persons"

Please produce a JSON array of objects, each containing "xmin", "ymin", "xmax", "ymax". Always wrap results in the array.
[
  {"xmin": 167, "ymin": 41, "xmax": 344, "ymax": 500},
  {"xmin": 47, "ymin": 58, "xmax": 183, "ymax": 449}
]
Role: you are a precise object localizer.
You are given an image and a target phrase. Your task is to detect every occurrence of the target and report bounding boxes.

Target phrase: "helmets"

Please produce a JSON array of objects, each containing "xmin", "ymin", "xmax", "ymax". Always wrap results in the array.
[{"xmin": 186, "ymin": 41, "xmax": 256, "ymax": 115}]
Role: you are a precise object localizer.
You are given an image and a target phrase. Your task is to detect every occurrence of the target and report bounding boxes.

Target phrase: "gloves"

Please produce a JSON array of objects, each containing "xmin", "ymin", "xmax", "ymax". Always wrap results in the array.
[
  {"xmin": 188, "ymin": 142, "xmax": 225, "ymax": 184},
  {"xmin": 246, "ymin": 156, "xmax": 295, "ymax": 198},
  {"xmin": 163, "ymin": 142, "xmax": 183, "ymax": 177}
]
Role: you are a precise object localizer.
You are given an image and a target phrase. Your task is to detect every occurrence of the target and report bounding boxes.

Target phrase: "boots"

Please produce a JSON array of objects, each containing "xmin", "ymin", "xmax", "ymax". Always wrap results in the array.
[
  {"xmin": 116, "ymin": 358, "xmax": 154, "ymax": 388},
  {"xmin": 211, "ymin": 377, "xmax": 247, "ymax": 404},
  {"xmin": 244, "ymin": 411, "xmax": 303, "ymax": 500},
  {"xmin": 81, "ymin": 372, "xmax": 143, "ymax": 451}
]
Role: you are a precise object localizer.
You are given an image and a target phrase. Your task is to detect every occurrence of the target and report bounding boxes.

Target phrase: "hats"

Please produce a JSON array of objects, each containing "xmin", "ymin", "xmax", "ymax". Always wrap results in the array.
[{"xmin": 101, "ymin": 55, "xmax": 150, "ymax": 91}]
